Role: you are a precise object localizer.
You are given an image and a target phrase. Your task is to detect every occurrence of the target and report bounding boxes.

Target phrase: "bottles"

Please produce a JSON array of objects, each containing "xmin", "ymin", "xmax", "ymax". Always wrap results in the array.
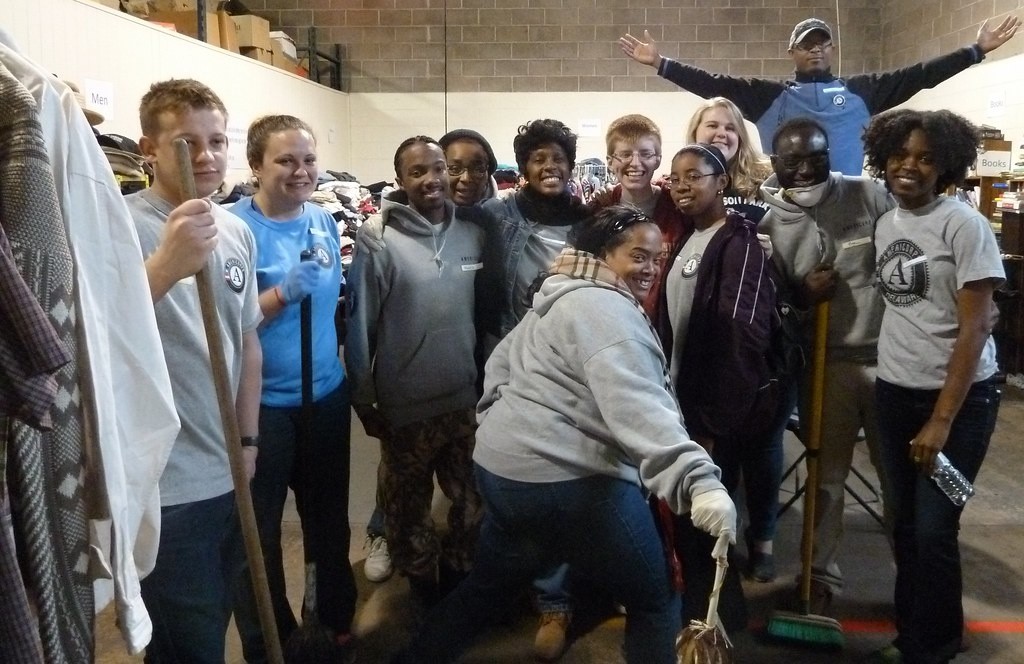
[{"xmin": 909, "ymin": 439, "xmax": 975, "ymax": 507}]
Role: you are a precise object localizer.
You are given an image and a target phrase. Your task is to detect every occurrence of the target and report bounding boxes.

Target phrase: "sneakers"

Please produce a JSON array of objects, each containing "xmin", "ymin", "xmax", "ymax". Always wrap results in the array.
[{"xmin": 362, "ymin": 533, "xmax": 394, "ymax": 582}]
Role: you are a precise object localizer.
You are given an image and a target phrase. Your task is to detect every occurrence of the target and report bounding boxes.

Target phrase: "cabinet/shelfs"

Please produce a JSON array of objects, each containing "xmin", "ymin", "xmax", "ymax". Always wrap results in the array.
[{"xmin": 948, "ymin": 139, "xmax": 1012, "ymax": 219}]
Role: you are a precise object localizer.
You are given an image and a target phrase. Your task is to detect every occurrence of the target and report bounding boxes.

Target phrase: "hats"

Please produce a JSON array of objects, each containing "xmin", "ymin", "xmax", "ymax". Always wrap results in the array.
[
  {"xmin": 787, "ymin": 17, "xmax": 833, "ymax": 52},
  {"xmin": 438, "ymin": 128, "xmax": 497, "ymax": 175}
]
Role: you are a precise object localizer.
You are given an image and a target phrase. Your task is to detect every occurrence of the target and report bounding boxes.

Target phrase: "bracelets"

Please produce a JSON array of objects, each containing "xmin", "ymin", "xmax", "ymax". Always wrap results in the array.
[
  {"xmin": 241, "ymin": 436, "xmax": 262, "ymax": 448},
  {"xmin": 275, "ymin": 289, "xmax": 284, "ymax": 305}
]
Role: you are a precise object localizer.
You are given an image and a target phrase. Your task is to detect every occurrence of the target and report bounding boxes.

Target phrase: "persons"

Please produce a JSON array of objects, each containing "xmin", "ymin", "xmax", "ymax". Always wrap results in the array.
[
  {"xmin": 124, "ymin": 77, "xmax": 265, "ymax": 664},
  {"xmin": 227, "ymin": 116, "xmax": 357, "ymax": 648},
  {"xmin": 356, "ymin": 96, "xmax": 798, "ymax": 628},
  {"xmin": 861, "ymin": 109, "xmax": 1006, "ymax": 664},
  {"xmin": 620, "ymin": 16, "xmax": 1021, "ymax": 175},
  {"xmin": 758, "ymin": 119, "xmax": 999, "ymax": 615},
  {"xmin": 345, "ymin": 139, "xmax": 489, "ymax": 603},
  {"xmin": 412, "ymin": 206, "xmax": 738, "ymax": 664}
]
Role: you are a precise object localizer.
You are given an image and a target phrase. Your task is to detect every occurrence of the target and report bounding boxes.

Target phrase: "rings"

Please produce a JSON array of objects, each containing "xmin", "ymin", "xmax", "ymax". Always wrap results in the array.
[{"xmin": 914, "ymin": 456, "xmax": 920, "ymax": 462}]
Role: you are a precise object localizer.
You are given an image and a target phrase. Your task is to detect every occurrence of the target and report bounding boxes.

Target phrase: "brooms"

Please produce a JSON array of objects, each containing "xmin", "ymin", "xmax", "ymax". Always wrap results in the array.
[{"xmin": 764, "ymin": 300, "xmax": 845, "ymax": 649}]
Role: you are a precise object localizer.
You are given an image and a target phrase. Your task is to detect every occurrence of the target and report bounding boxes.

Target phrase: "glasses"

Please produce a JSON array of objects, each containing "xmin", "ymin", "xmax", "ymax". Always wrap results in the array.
[
  {"xmin": 774, "ymin": 152, "xmax": 830, "ymax": 169},
  {"xmin": 609, "ymin": 151, "xmax": 659, "ymax": 161},
  {"xmin": 447, "ymin": 163, "xmax": 492, "ymax": 177},
  {"xmin": 669, "ymin": 172, "xmax": 717, "ymax": 188},
  {"xmin": 792, "ymin": 34, "xmax": 832, "ymax": 50}
]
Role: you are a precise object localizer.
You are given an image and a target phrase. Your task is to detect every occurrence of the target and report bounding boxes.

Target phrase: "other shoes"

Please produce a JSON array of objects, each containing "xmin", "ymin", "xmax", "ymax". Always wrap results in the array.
[
  {"xmin": 866, "ymin": 642, "xmax": 904, "ymax": 664},
  {"xmin": 742, "ymin": 525, "xmax": 774, "ymax": 582},
  {"xmin": 795, "ymin": 579, "xmax": 831, "ymax": 618},
  {"xmin": 311, "ymin": 628, "xmax": 356, "ymax": 664},
  {"xmin": 535, "ymin": 607, "xmax": 575, "ymax": 661}
]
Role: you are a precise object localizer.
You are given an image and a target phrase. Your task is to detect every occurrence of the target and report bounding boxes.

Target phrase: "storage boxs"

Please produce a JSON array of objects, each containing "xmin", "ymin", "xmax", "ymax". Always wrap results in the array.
[{"xmin": 145, "ymin": 11, "xmax": 310, "ymax": 79}]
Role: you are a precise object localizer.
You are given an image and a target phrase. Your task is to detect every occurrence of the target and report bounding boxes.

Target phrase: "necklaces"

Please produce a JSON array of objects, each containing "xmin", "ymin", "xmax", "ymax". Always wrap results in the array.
[{"xmin": 623, "ymin": 197, "xmax": 650, "ymax": 205}]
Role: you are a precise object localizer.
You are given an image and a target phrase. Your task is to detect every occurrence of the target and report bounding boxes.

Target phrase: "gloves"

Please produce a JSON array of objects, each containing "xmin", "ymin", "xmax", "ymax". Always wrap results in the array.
[
  {"xmin": 280, "ymin": 261, "xmax": 321, "ymax": 305},
  {"xmin": 690, "ymin": 489, "xmax": 736, "ymax": 558},
  {"xmin": 353, "ymin": 214, "xmax": 386, "ymax": 255}
]
